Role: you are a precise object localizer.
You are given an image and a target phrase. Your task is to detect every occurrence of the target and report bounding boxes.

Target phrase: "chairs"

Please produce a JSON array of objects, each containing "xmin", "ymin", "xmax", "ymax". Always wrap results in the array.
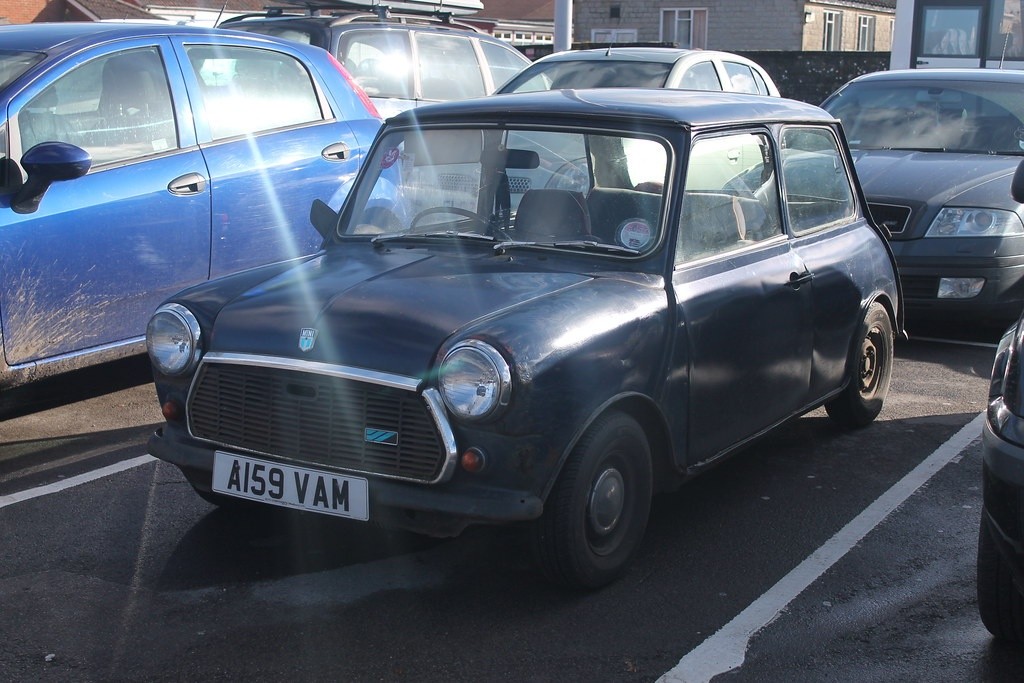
[
  {"xmin": 515, "ymin": 189, "xmax": 591, "ymax": 243},
  {"xmin": 679, "ymin": 192, "xmax": 765, "ymax": 261},
  {"xmin": 95, "ymin": 51, "xmax": 164, "ymax": 145}
]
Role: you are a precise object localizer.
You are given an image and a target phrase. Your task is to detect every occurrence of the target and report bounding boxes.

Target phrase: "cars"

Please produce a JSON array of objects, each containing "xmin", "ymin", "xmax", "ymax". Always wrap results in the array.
[
  {"xmin": 0, "ymin": 20, "xmax": 414, "ymax": 393},
  {"xmin": 142, "ymin": 88, "xmax": 911, "ymax": 603},
  {"xmin": 722, "ymin": 67, "xmax": 1024, "ymax": 349},
  {"xmin": 403, "ymin": 45, "xmax": 788, "ymax": 233},
  {"xmin": 977, "ymin": 158, "xmax": 1024, "ymax": 646}
]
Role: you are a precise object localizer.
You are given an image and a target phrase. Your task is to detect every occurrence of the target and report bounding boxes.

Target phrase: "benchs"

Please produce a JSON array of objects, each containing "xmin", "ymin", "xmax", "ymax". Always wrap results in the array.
[{"xmin": 586, "ymin": 187, "xmax": 662, "ymax": 253}]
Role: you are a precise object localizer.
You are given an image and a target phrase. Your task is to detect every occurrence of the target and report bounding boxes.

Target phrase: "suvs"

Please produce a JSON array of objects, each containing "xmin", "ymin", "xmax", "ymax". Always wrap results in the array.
[{"xmin": 191, "ymin": 1, "xmax": 557, "ymax": 154}]
